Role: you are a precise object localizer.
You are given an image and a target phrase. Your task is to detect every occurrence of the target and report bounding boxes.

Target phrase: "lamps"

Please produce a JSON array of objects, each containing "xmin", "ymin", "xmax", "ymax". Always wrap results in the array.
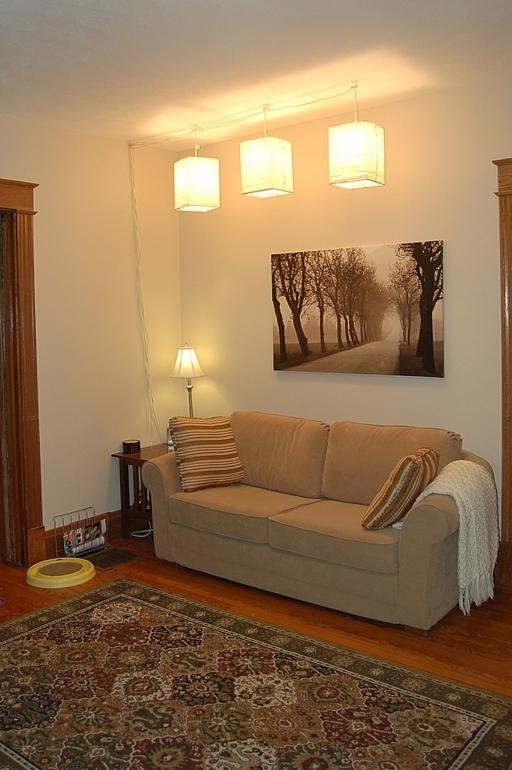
[
  {"xmin": 169, "ymin": 82, "xmax": 387, "ymax": 215},
  {"xmin": 169, "ymin": 341, "xmax": 204, "ymax": 418}
]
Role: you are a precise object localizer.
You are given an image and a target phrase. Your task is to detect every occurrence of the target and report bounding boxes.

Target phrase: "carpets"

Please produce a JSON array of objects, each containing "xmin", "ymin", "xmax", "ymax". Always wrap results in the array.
[{"xmin": 2, "ymin": 574, "xmax": 511, "ymax": 770}]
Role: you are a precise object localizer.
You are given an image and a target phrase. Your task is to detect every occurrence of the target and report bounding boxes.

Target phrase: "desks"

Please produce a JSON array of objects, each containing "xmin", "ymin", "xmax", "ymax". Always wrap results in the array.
[{"xmin": 111, "ymin": 444, "xmax": 171, "ymax": 539}]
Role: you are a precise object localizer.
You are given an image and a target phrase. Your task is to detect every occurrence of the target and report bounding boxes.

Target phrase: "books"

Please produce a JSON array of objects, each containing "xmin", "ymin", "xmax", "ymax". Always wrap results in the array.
[{"xmin": 63, "ymin": 519, "xmax": 107, "ymax": 555}]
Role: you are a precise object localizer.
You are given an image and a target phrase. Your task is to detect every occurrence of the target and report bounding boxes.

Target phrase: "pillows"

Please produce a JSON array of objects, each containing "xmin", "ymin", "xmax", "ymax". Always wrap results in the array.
[
  {"xmin": 169, "ymin": 416, "xmax": 246, "ymax": 494},
  {"xmin": 360, "ymin": 447, "xmax": 440, "ymax": 531}
]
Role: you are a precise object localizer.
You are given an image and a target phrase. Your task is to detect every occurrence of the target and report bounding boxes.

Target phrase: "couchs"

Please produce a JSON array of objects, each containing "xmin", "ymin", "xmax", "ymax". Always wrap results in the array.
[{"xmin": 140, "ymin": 410, "xmax": 503, "ymax": 634}]
